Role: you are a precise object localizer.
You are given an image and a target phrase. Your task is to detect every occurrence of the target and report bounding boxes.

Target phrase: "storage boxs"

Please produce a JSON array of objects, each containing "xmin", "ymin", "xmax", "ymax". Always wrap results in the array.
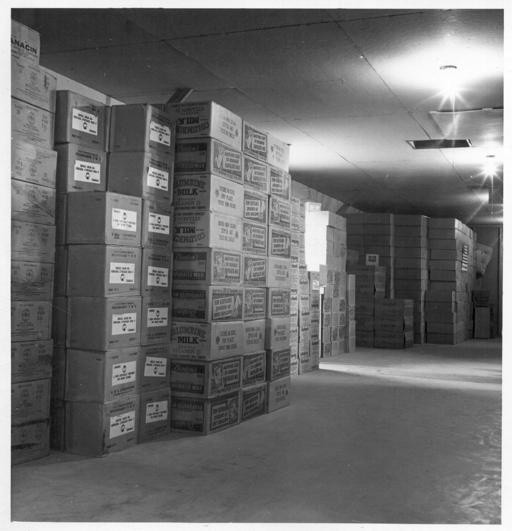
[{"xmin": 307, "ymin": 198, "xmax": 495, "ymax": 357}]
